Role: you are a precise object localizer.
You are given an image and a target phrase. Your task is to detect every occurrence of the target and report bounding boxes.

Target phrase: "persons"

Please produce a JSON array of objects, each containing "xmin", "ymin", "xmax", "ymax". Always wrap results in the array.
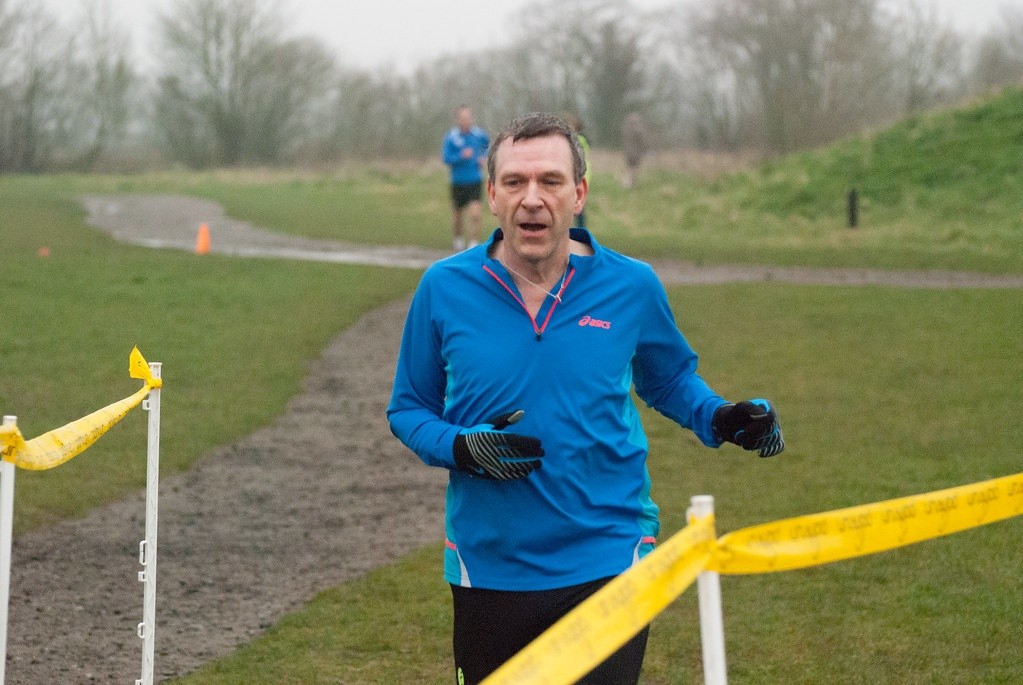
[
  {"xmin": 623, "ymin": 105, "xmax": 648, "ymax": 191},
  {"xmin": 569, "ymin": 118, "xmax": 591, "ymax": 228},
  {"xmin": 384, "ymin": 112, "xmax": 787, "ymax": 685},
  {"xmin": 441, "ymin": 104, "xmax": 492, "ymax": 251}
]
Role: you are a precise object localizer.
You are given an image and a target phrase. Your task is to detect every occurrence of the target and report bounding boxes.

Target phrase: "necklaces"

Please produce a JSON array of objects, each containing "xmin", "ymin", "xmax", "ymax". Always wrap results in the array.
[{"xmin": 501, "ymin": 258, "xmax": 568, "ymax": 306}]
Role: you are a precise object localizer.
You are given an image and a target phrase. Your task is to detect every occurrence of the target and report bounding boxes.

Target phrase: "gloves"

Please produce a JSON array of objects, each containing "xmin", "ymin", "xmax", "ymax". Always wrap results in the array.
[
  {"xmin": 712, "ymin": 398, "xmax": 786, "ymax": 458},
  {"xmin": 453, "ymin": 410, "xmax": 546, "ymax": 482}
]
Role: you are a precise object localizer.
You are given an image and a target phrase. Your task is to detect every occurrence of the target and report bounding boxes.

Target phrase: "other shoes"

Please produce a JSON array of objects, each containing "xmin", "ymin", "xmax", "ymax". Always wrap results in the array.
[
  {"xmin": 452, "ymin": 236, "xmax": 466, "ymax": 253},
  {"xmin": 467, "ymin": 240, "xmax": 479, "ymax": 249}
]
